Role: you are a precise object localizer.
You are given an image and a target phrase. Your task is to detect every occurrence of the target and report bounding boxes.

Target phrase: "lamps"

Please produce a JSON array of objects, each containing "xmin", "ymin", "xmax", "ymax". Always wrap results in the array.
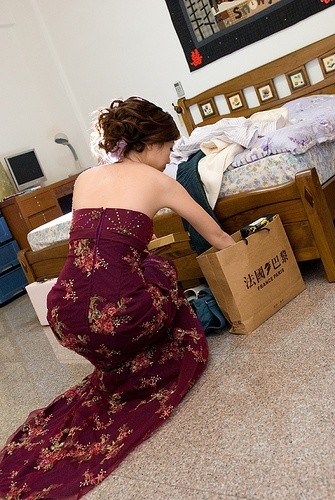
[{"xmin": 53, "ymin": 132, "xmax": 90, "ymax": 178}]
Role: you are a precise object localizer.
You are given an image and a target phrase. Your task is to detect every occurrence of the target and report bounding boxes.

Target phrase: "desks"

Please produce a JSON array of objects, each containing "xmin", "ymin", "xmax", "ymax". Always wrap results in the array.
[{"xmin": 0, "ymin": 166, "xmax": 91, "ymax": 250}]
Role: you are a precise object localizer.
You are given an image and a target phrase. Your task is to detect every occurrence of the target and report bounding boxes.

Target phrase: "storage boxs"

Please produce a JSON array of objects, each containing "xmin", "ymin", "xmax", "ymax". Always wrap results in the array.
[{"xmin": 25, "ymin": 275, "xmax": 60, "ymax": 327}]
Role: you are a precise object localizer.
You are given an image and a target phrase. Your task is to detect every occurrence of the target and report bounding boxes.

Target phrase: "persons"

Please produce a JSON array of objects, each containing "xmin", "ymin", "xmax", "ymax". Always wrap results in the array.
[{"xmin": 0, "ymin": 96, "xmax": 235, "ymax": 500}]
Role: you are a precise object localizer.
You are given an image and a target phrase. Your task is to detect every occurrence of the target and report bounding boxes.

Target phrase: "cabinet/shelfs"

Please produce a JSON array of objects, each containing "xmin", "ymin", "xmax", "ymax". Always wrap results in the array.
[
  {"xmin": 0, "ymin": 217, "xmax": 30, "ymax": 307},
  {"xmin": 0, "ymin": 189, "xmax": 64, "ymax": 252}
]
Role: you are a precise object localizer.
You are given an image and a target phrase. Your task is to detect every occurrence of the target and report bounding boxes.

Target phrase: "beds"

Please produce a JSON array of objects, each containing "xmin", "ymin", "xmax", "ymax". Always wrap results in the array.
[{"xmin": 16, "ymin": 32, "xmax": 335, "ymax": 286}]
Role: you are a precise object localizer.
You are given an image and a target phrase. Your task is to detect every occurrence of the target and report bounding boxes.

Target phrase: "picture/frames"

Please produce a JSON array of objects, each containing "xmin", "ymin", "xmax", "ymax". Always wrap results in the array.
[
  {"xmin": 253, "ymin": 79, "xmax": 279, "ymax": 107},
  {"xmin": 224, "ymin": 89, "xmax": 248, "ymax": 114},
  {"xmin": 285, "ymin": 64, "xmax": 311, "ymax": 95},
  {"xmin": 197, "ymin": 96, "xmax": 220, "ymax": 122},
  {"xmin": 164, "ymin": 0, "xmax": 335, "ymax": 73},
  {"xmin": 317, "ymin": 48, "xmax": 335, "ymax": 79}
]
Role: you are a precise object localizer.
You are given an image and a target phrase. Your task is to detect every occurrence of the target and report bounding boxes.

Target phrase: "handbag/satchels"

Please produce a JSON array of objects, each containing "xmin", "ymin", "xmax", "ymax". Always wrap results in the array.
[
  {"xmin": 184, "ymin": 284, "xmax": 226, "ymax": 334},
  {"xmin": 196, "ymin": 212, "xmax": 307, "ymax": 335}
]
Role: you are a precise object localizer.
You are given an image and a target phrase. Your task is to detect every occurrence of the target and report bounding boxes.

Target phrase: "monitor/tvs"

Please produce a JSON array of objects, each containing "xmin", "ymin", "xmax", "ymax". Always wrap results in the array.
[{"xmin": 4, "ymin": 146, "xmax": 48, "ymax": 191}]
